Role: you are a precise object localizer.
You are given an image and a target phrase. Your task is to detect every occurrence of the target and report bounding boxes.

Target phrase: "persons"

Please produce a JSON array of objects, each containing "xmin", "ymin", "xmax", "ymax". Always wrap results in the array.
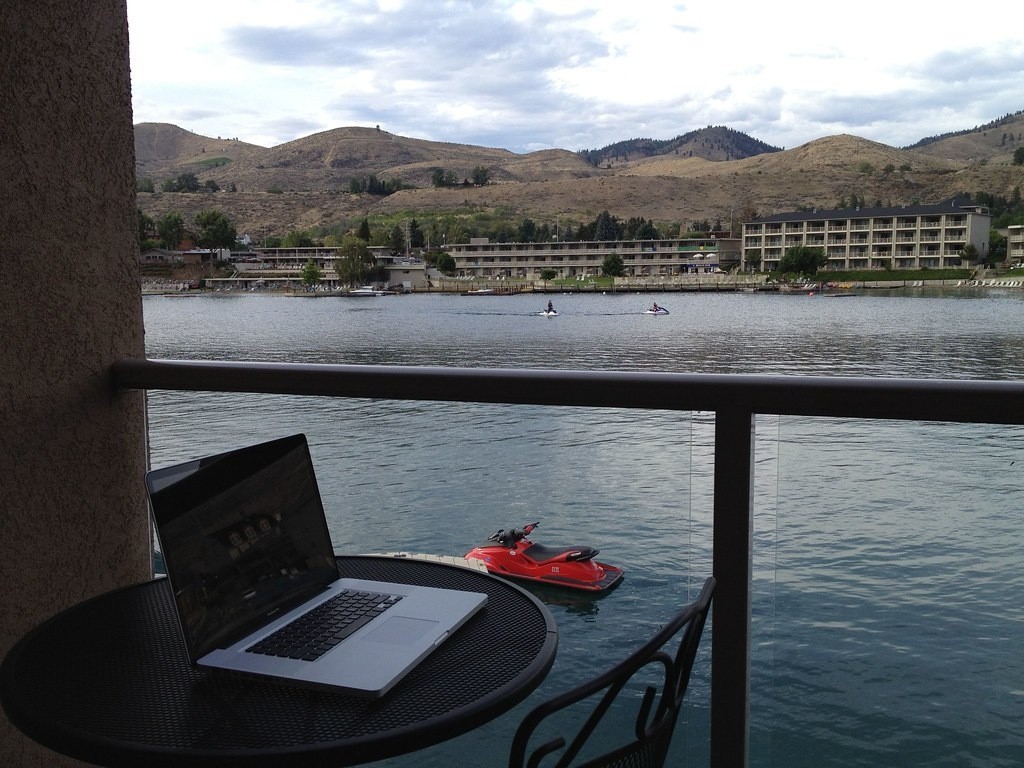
[
  {"xmin": 548, "ymin": 300, "xmax": 553, "ymax": 312},
  {"xmin": 654, "ymin": 303, "xmax": 657, "ymax": 311}
]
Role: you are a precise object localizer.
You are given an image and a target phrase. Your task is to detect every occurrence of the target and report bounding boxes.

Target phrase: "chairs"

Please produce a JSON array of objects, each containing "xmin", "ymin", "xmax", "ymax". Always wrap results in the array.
[{"xmin": 508, "ymin": 577, "xmax": 715, "ymax": 768}]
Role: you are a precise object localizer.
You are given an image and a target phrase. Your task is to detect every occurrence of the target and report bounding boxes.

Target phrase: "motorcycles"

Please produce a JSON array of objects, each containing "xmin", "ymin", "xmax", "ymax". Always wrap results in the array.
[
  {"xmin": 460, "ymin": 519, "xmax": 625, "ymax": 598},
  {"xmin": 539, "ymin": 302, "xmax": 559, "ymax": 317},
  {"xmin": 642, "ymin": 302, "xmax": 670, "ymax": 316}
]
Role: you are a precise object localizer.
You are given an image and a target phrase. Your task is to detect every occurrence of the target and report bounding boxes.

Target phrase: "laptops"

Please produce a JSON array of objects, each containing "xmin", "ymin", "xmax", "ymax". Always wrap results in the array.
[{"xmin": 144, "ymin": 432, "xmax": 489, "ymax": 697}]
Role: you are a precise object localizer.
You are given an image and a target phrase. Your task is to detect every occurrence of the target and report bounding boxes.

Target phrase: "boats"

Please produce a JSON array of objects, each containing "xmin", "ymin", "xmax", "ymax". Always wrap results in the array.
[{"xmin": 349, "ymin": 286, "xmax": 387, "ymax": 296}]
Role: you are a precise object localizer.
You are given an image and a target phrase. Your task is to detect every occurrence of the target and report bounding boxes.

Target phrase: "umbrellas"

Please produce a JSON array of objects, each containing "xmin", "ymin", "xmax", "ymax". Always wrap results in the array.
[
  {"xmin": 706, "ymin": 253, "xmax": 716, "ymax": 260},
  {"xmin": 693, "ymin": 254, "xmax": 704, "ymax": 260}
]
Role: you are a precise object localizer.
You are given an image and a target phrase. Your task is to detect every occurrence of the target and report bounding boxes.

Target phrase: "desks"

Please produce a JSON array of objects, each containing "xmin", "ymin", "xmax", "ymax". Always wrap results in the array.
[{"xmin": 0, "ymin": 557, "xmax": 556, "ymax": 768}]
